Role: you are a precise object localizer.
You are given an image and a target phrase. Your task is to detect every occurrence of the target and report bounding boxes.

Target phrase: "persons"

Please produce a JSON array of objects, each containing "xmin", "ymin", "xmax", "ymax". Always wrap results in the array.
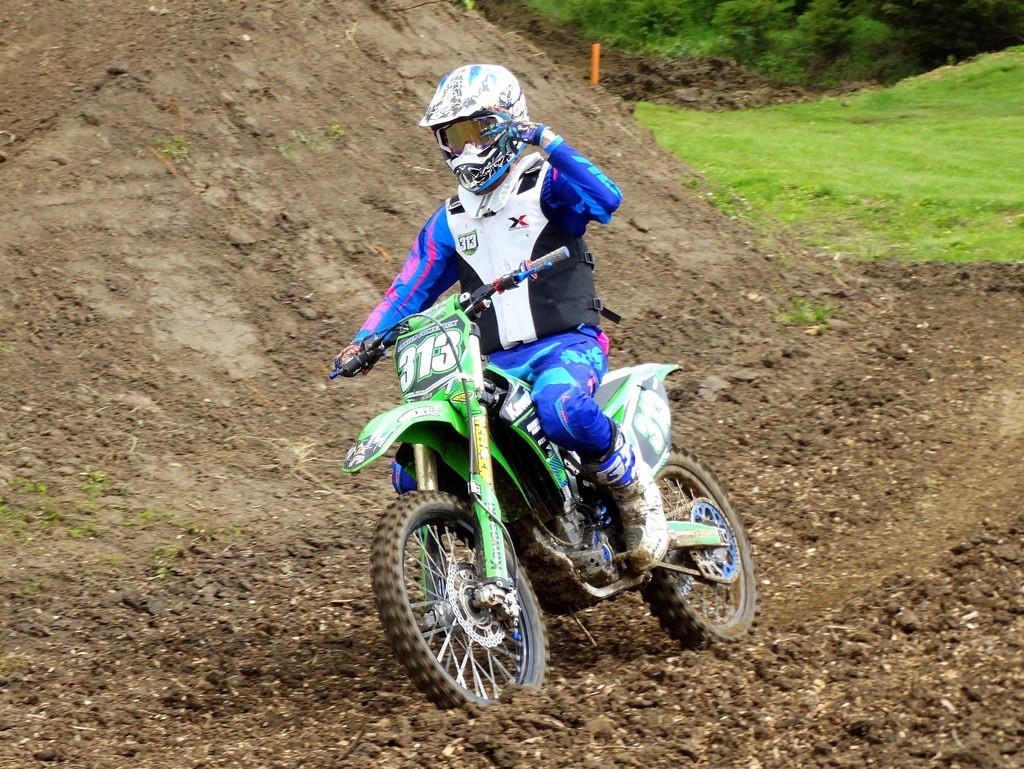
[{"xmin": 333, "ymin": 64, "xmax": 671, "ymax": 573}]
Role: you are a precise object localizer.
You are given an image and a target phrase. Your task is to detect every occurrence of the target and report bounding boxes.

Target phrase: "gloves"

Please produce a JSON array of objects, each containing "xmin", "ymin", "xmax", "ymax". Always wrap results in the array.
[
  {"xmin": 479, "ymin": 104, "xmax": 552, "ymax": 155},
  {"xmin": 334, "ymin": 341, "xmax": 379, "ymax": 377}
]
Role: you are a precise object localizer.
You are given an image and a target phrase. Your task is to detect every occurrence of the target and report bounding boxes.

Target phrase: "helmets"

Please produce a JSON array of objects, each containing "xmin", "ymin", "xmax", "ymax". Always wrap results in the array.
[{"xmin": 419, "ymin": 63, "xmax": 530, "ymax": 194}]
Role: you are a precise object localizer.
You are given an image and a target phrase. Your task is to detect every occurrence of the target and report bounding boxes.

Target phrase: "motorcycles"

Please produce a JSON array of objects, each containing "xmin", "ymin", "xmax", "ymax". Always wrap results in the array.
[{"xmin": 329, "ymin": 247, "xmax": 759, "ymax": 708}]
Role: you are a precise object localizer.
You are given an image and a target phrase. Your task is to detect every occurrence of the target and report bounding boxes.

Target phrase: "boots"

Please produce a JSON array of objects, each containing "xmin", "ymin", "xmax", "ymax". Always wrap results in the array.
[{"xmin": 580, "ymin": 415, "xmax": 670, "ymax": 573}]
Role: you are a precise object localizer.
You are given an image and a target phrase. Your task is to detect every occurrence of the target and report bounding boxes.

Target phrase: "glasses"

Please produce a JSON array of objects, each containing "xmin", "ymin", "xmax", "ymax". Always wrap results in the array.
[{"xmin": 433, "ymin": 87, "xmax": 527, "ymax": 157}]
